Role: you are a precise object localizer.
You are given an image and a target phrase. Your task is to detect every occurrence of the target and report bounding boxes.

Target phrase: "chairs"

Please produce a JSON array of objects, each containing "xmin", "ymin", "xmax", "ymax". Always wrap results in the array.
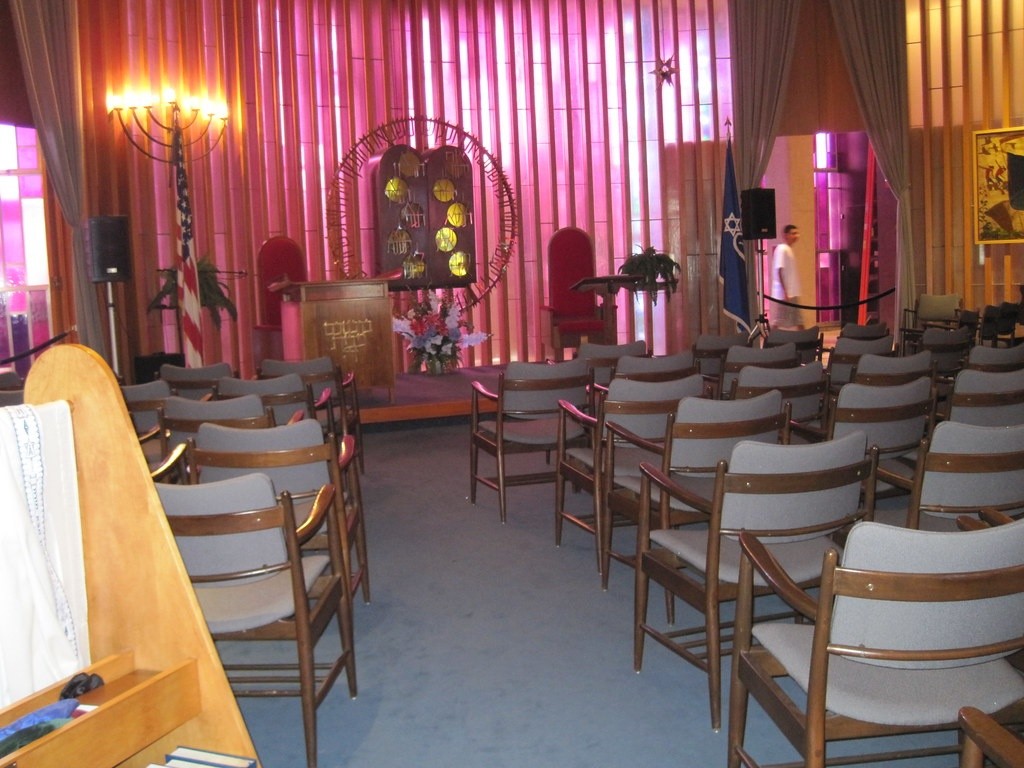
[
  {"xmin": 115, "ymin": 357, "xmax": 372, "ymax": 767},
  {"xmin": 468, "ymin": 354, "xmax": 600, "ymax": 526},
  {"xmin": 540, "ymin": 225, "xmax": 618, "ymax": 363},
  {"xmin": 256, "ymin": 236, "xmax": 312, "ymax": 357},
  {"xmin": 545, "ymin": 290, "xmax": 1023, "ymax": 768}
]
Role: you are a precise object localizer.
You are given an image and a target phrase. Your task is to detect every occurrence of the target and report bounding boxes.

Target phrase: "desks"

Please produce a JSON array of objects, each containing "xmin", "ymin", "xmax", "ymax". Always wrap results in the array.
[{"xmin": 268, "ymin": 265, "xmax": 404, "ymax": 404}]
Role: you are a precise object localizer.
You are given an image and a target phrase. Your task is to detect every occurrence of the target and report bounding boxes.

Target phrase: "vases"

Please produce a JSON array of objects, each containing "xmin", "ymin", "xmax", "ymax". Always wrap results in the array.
[{"xmin": 424, "ymin": 356, "xmax": 445, "ymax": 375}]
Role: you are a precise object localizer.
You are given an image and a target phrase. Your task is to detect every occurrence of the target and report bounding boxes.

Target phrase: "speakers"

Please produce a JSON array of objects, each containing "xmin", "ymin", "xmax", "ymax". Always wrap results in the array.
[
  {"xmin": 740, "ymin": 188, "xmax": 777, "ymax": 240},
  {"xmin": 135, "ymin": 352, "xmax": 185, "ymax": 385},
  {"xmin": 87, "ymin": 215, "xmax": 132, "ymax": 283}
]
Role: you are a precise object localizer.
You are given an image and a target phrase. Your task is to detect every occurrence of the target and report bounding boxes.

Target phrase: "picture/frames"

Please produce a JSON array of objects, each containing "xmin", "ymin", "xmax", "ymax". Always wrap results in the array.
[{"xmin": 972, "ymin": 125, "xmax": 1024, "ymax": 245}]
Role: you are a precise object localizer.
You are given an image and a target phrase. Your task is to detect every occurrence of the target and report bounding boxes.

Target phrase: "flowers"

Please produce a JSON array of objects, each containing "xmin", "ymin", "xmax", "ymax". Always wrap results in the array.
[{"xmin": 392, "ymin": 276, "xmax": 493, "ymax": 375}]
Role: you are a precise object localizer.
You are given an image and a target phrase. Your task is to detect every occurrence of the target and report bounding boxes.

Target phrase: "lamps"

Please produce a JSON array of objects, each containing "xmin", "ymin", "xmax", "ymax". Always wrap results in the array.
[{"xmin": 106, "ymin": 85, "xmax": 232, "ymax": 166}]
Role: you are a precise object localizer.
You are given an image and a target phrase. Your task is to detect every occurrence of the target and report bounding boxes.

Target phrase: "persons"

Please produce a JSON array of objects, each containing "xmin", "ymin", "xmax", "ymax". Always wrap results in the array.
[{"xmin": 770, "ymin": 225, "xmax": 806, "ymax": 331}]
